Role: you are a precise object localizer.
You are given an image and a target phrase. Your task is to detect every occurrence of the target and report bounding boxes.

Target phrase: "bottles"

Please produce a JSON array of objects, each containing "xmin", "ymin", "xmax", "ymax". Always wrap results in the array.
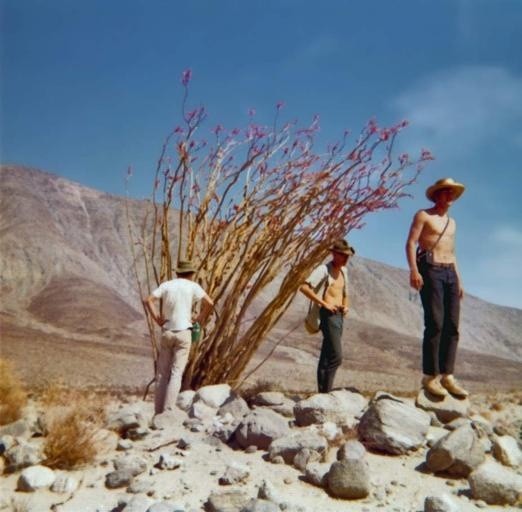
[{"xmin": 192, "ymin": 321, "xmax": 201, "ymax": 339}]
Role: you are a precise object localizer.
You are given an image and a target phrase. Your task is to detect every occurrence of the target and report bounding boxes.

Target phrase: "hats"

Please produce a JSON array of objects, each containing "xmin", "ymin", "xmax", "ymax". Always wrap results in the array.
[
  {"xmin": 426, "ymin": 178, "xmax": 464, "ymax": 203},
  {"xmin": 172, "ymin": 261, "xmax": 196, "ymax": 273},
  {"xmin": 327, "ymin": 240, "xmax": 354, "ymax": 255}
]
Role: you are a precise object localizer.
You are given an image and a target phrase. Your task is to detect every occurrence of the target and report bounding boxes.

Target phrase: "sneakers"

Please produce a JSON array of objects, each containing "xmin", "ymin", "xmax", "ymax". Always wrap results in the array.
[
  {"xmin": 441, "ymin": 377, "xmax": 469, "ymax": 396},
  {"xmin": 422, "ymin": 377, "xmax": 448, "ymax": 396}
]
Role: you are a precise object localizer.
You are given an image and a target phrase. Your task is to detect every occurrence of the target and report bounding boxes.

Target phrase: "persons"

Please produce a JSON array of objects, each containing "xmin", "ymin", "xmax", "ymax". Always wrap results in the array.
[
  {"xmin": 141, "ymin": 260, "xmax": 214, "ymax": 414},
  {"xmin": 405, "ymin": 177, "xmax": 469, "ymax": 398},
  {"xmin": 299, "ymin": 238, "xmax": 355, "ymax": 392}
]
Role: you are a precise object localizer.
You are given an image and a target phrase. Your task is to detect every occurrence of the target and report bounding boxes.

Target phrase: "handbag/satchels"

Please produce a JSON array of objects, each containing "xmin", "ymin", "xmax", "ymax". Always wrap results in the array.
[{"xmin": 417, "ymin": 250, "xmax": 433, "ymax": 269}]
